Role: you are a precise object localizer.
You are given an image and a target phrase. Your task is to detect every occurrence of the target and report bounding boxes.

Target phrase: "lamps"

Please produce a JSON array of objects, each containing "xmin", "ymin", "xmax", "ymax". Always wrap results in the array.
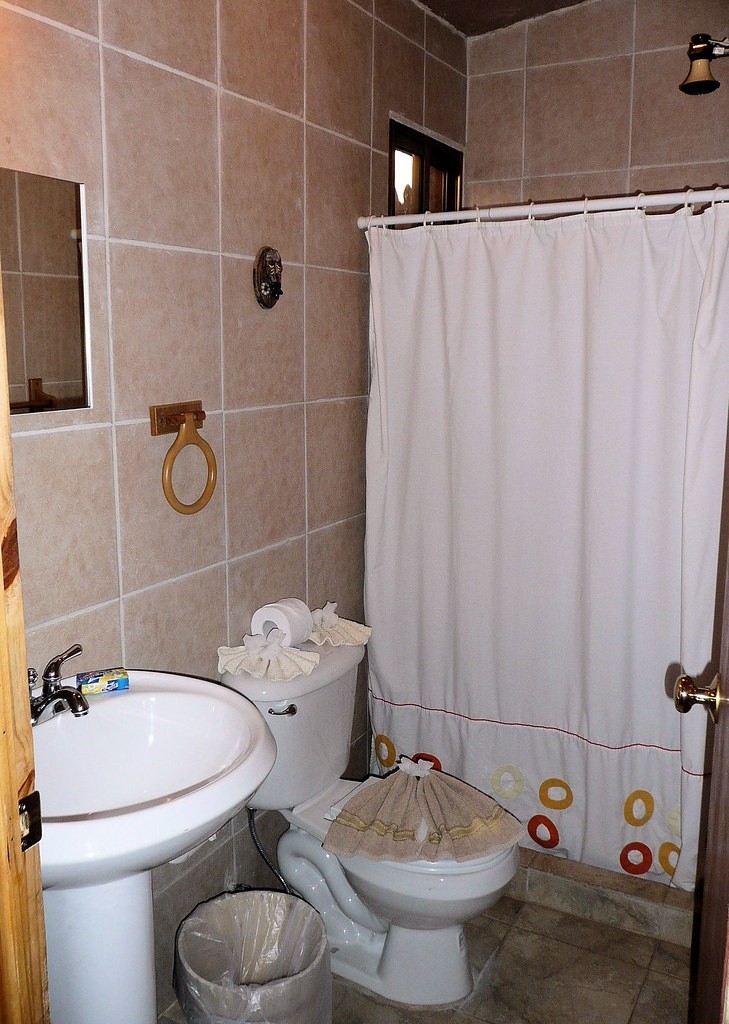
[{"xmin": 678, "ymin": 32, "xmax": 729, "ymax": 96}]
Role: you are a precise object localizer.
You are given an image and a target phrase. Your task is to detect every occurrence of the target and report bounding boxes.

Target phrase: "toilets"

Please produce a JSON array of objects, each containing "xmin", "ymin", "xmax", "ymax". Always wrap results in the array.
[{"xmin": 220, "ymin": 622, "xmax": 526, "ymax": 1006}]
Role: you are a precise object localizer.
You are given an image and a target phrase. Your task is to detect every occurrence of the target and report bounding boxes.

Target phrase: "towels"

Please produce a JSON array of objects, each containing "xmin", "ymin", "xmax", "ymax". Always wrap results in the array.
[{"xmin": 322, "ymin": 755, "xmax": 527, "ymax": 864}]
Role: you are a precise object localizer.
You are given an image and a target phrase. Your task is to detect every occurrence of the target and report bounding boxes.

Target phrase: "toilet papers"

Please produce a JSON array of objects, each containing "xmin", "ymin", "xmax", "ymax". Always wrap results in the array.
[{"xmin": 250, "ymin": 597, "xmax": 313, "ymax": 647}]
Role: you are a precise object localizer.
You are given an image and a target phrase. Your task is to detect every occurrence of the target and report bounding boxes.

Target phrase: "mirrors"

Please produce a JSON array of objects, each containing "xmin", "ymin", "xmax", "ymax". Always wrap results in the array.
[{"xmin": 0, "ymin": 167, "xmax": 90, "ymax": 416}]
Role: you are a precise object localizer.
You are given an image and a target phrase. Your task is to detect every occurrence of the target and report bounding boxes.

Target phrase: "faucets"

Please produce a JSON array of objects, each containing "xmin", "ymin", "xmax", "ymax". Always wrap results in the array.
[{"xmin": 28, "ymin": 643, "xmax": 90, "ymax": 728}]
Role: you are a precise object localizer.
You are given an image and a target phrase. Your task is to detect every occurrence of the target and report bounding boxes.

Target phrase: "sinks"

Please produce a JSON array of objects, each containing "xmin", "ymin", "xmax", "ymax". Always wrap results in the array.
[{"xmin": 28, "ymin": 670, "xmax": 279, "ymax": 883}]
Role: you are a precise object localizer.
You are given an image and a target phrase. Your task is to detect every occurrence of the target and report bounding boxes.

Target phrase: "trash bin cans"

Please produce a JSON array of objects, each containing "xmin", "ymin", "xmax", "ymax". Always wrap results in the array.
[{"xmin": 176, "ymin": 891, "xmax": 328, "ymax": 1024}]
